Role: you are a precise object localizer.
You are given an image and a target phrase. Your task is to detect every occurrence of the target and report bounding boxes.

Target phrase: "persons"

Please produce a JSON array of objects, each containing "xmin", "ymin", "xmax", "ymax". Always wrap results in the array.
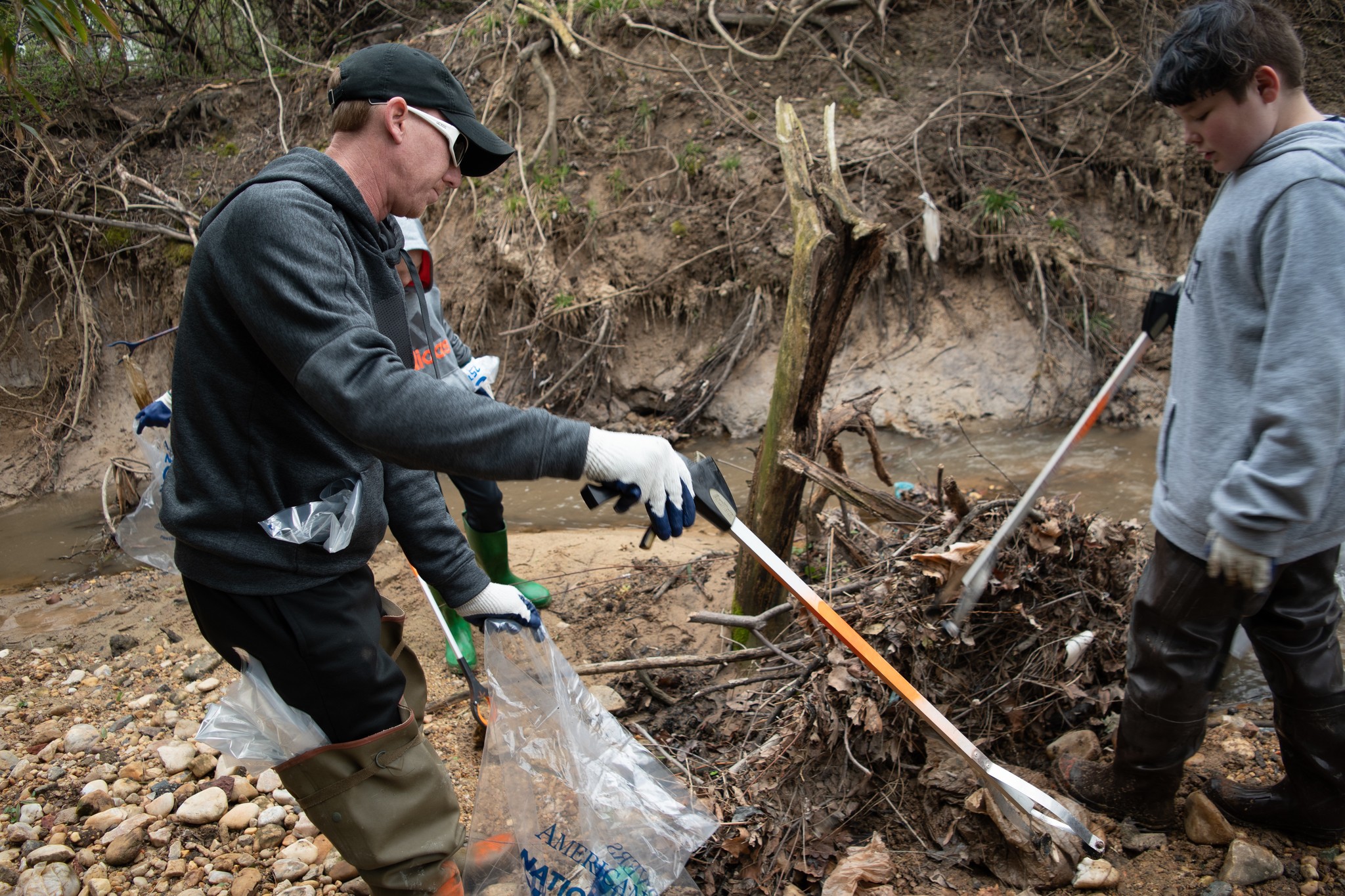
[
  {"xmin": 161, "ymin": 42, "xmax": 697, "ymax": 896},
  {"xmin": 391, "ymin": 213, "xmax": 553, "ymax": 675},
  {"xmin": 1051, "ymin": 0, "xmax": 1345, "ymax": 847}
]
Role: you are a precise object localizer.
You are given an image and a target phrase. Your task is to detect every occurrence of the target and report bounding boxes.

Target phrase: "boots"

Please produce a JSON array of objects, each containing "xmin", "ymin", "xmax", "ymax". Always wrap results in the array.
[
  {"xmin": 374, "ymin": 596, "xmax": 519, "ymax": 889},
  {"xmin": 428, "ymin": 582, "xmax": 478, "ymax": 673},
  {"xmin": 1205, "ymin": 700, "xmax": 1345, "ymax": 845},
  {"xmin": 462, "ymin": 512, "xmax": 552, "ymax": 609},
  {"xmin": 1052, "ymin": 684, "xmax": 1211, "ymax": 830},
  {"xmin": 268, "ymin": 701, "xmax": 466, "ymax": 896}
]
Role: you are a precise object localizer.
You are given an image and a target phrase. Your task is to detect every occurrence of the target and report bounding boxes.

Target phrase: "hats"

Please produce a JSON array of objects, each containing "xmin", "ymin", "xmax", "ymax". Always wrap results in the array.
[{"xmin": 326, "ymin": 44, "xmax": 516, "ymax": 177}]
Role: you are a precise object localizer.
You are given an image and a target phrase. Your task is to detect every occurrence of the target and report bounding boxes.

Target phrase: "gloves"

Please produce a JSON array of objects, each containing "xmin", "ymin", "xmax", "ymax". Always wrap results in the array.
[
  {"xmin": 1202, "ymin": 528, "xmax": 1273, "ymax": 591},
  {"xmin": 459, "ymin": 357, "xmax": 495, "ymax": 400},
  {"xmin": 583, "ymin": 426, "xmax": 695, "ymax": 540},
  {"xmin": 452, "ymin": 582, "xmax": 546, "ymax": 644},
  {"xmin": 135, "ymin": 390, "xmax": 173, "ymax": 434}
]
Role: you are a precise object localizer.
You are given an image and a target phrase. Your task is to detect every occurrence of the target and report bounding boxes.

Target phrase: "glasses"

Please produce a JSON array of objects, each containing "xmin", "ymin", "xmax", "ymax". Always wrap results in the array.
[{"xmin": 366, "ymin": 96, "xmax": 468, "ymax": 171}]
